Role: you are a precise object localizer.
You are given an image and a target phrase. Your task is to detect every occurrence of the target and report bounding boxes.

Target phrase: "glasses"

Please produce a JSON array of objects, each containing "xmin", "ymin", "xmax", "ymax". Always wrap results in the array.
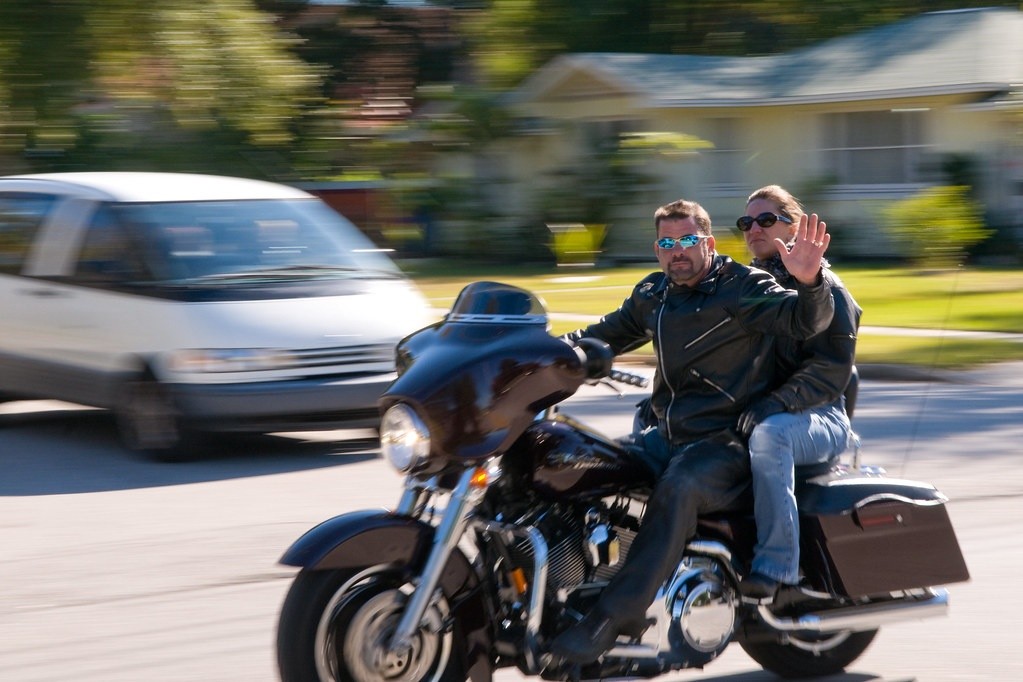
[
  {"xmin": 736, "ymin": 212, "xmax": 791, "ymax": 231},
  {"xmin": 656, "ymin": 233, "xmax": 709, "ymax": 250}
]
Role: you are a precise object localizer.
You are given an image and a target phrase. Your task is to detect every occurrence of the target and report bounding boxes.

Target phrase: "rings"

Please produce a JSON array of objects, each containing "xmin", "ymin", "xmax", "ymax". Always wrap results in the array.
[{"xmin": 814, "ymin": 241, "xmax": 821, "ymax": 247}]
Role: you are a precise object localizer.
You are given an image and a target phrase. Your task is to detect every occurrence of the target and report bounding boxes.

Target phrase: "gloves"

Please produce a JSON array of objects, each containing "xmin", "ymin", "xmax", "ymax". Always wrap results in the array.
[
  {"xmin": 636, "ymin": 398, "xmax": 658, "ymax": 421},
  {"xmin": 736, "ymin": 397, "xmax": 786, "ymax": 435}
]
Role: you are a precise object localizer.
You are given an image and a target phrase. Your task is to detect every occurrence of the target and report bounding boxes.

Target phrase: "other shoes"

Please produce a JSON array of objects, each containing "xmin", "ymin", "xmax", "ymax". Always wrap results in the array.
[{"xmin": 738, "ymin": 568, "xmax": 797, "ymax": 605}]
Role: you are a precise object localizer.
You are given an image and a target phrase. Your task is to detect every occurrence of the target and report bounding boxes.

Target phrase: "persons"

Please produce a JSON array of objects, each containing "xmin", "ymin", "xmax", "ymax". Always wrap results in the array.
[
  {"xmin": 550, "ymin": 198, "xmax": 835, "ymax": 664},
  {"xmin": 737, "ymin": 184, "xmax": 864, "ymax": 604}
]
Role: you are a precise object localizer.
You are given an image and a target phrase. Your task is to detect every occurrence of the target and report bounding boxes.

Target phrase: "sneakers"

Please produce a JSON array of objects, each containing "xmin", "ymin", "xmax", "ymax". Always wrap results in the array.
[{"xmin": 560, "ymin": 609, "xmax": 622, "ymax": 664}]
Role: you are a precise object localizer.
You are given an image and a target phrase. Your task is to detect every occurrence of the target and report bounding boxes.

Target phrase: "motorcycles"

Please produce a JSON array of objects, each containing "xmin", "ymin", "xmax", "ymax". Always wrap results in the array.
[{"xmin": 275, "ymin": 278, "xmax": 972, "ymax": 682}]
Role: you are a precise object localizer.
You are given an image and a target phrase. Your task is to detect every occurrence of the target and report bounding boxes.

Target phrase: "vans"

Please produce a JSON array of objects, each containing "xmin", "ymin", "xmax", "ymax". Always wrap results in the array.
[{"xmin": 0, "ymin": 170, "xmax": 449, "ymax": 464}]
